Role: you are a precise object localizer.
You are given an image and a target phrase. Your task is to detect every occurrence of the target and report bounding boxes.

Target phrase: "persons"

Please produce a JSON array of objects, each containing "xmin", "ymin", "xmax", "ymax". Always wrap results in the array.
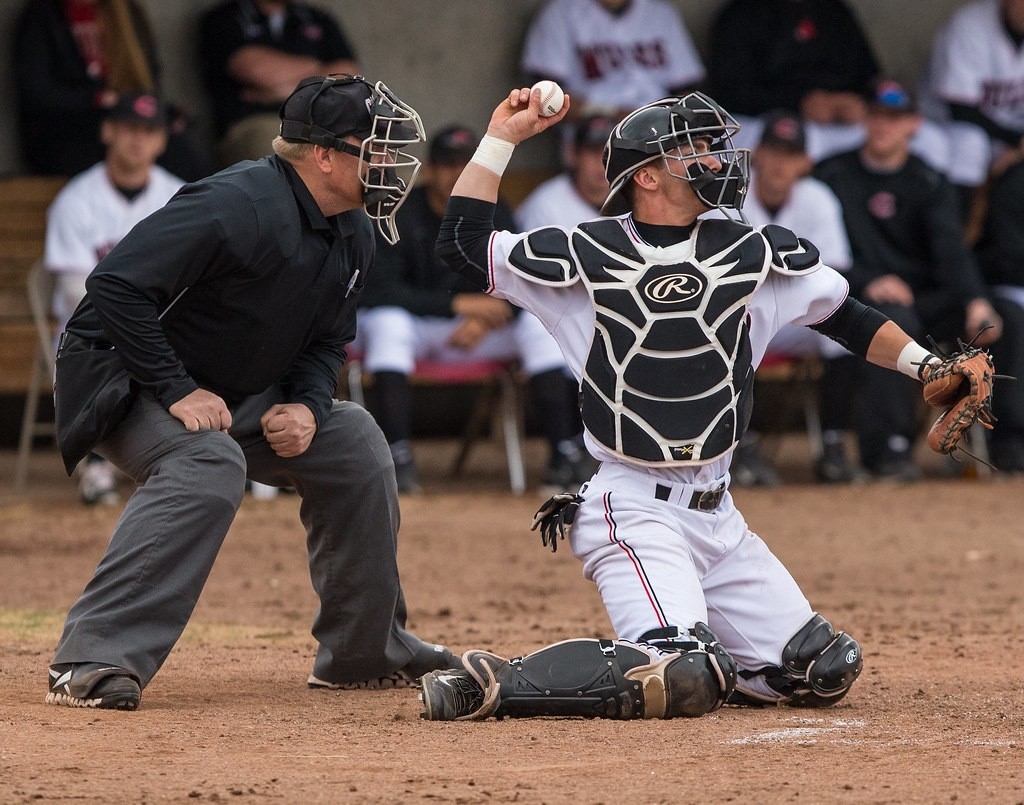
[
  {"xmin": 43, "ymin": 72, "xmax": 463, "ymax": 710},
  {"xmin": 415, "ymin": 88, "xmax": 1014, "ymax": 715},
  {"xmin": 11, "ymin": 0, "xmax": 1024, "ymax": 508}
]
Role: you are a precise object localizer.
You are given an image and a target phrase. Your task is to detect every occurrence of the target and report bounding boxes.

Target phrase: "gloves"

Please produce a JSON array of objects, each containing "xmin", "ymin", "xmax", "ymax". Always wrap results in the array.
[{"xmin": 532, "ymin": 493, "xmax": 585, "ymax": 552}]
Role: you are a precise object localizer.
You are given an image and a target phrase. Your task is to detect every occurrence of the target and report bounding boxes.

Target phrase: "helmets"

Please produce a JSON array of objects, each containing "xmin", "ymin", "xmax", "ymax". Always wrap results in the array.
[{"xmin": 600, "ymin": 105, "xmax": 712, "ymax": 216}]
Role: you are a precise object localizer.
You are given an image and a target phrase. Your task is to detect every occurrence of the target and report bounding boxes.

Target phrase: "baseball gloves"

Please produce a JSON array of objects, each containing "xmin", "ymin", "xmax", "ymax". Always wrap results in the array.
[{"xmin": 924, "ymin": 349, "xmax": 995, "ymax": 453}]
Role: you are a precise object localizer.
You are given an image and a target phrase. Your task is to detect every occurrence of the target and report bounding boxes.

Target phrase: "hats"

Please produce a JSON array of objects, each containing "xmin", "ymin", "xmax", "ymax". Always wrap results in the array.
[
  {"xmin": 577, "ymin": 116, "xmax": 616, "ymax": 144},
  {"xmin": 429, "ymin": 122, "xmax": 480, "ymax": 164},
  {"xmin": 280, "ymin": 76, "xmax": 415, "ymax": 147},
  {"xmin": 760, "ymin": 116, "xmax": 806, "ymax": 154},
  {"xmin": 869, "ymin": 83, "xmax": 915, "ymax": 117},
  {"xmin": 109, "ymin": 93, "xmax": 168, "ymax": 126}
]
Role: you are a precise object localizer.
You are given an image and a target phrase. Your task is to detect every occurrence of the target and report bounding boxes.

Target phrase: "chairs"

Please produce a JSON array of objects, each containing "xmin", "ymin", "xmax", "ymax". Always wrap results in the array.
[
  {"xmin": 754, "ymin": 355, "xmax": 825, "ymax": 478},
  {"xmin": 340, "ymin": 352, "xmax": 524, "ymax": 496},
  {"xmin": 12, "ymin": 254, "xmax": 74, "ymax": 486}
]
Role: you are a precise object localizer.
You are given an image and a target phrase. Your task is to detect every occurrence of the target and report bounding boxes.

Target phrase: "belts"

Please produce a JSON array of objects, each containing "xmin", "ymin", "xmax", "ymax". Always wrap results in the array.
[{"xmin": 654, "ymin": 482, "xmax": 725, "ymax": 512}]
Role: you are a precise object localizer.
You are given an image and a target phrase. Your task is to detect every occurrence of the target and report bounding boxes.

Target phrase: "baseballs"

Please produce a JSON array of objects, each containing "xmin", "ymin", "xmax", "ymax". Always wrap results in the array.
[{"xmin": 530, "ymin": 80, "xmax": 565, "ymax": 117}]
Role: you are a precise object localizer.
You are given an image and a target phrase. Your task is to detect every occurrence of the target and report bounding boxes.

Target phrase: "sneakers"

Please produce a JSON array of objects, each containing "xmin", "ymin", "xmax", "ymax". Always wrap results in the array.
[
  {"xmin": 46, "ymin": 661, "xmax": 141, "ymax": 710},
  {"xmin": 308, "ymin": 640, "xmax": 463, "ymax": 689},
  {"xmin": 418, "ymin": 664, "xmax": 469, "ymax": 718}
]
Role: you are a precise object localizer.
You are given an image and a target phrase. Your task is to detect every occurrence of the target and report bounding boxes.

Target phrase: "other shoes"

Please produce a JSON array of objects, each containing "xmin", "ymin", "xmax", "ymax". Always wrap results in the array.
[
  {"xmin": 813, "ymin": 452, "xmax": 852, "ymax": 484},
  {"xmin": 390, "ymin": 449, "xmax": 420, "ymax": 497},
  {"xmin": 730, "ymin": 454, "xmax": 762, "ymax": 486},
  {"xmin": 865, "ymin": 450, "xmax": 919, "ymax": 483},
  {"xmin": 543, "ymin": 454, "xmax": 593, "ymax": 494},
  {"xmin": 988, "ymin": 436, "xmax": 1024, "ymax": 471},
  {"xmin": 79, "ymin": 467, "xmax": 120, "ymax": 507}
]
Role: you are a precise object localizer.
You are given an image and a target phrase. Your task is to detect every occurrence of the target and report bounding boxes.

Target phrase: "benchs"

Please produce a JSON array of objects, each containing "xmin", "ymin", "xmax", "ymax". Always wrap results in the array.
[{"xmin": 0, "ymin": 168, "xmax": 553, "ymax": 392}]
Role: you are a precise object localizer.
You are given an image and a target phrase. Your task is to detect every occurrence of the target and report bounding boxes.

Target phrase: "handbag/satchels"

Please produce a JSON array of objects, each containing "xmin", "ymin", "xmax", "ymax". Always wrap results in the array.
[{"xmin": 56, "ymin": 342, "xmax": 130, "ymax": 477}]
